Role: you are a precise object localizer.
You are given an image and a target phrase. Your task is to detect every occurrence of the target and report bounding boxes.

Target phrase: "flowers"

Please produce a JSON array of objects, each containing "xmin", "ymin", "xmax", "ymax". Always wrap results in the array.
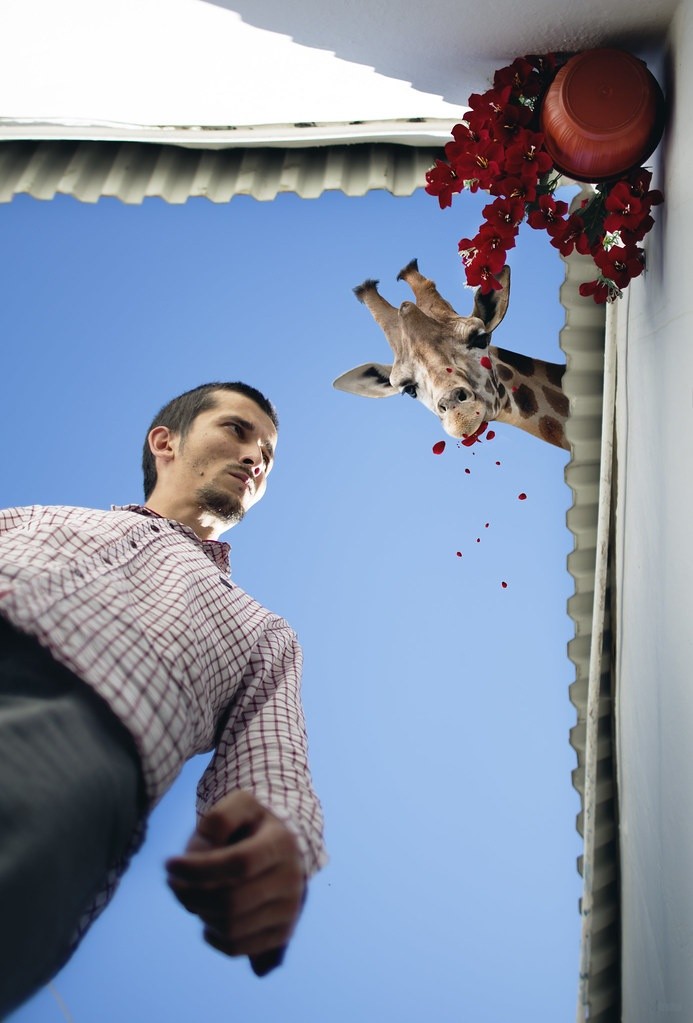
[{"xmin": 422, "ymin": 56, "xmax": 661, "ymax": 308}]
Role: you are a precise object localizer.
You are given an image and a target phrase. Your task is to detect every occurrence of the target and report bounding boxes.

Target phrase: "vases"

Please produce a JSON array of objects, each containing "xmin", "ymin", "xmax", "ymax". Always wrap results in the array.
[{"xmin": 538, "ymin": 47, "xmax": 669, "ymax": 182}]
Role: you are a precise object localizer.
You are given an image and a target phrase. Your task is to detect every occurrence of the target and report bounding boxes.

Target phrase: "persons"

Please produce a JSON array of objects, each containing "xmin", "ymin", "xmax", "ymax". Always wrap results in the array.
[{"xmin": 0, "ymin": 384, "xmax": 327, "ymax": 1023}]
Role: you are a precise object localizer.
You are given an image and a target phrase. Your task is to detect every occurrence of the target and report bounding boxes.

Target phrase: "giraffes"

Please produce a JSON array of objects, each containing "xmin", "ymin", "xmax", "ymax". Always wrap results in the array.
[{"xmin": 331, "ymin": 258, "xmax": 571, "ymax": 451}]
